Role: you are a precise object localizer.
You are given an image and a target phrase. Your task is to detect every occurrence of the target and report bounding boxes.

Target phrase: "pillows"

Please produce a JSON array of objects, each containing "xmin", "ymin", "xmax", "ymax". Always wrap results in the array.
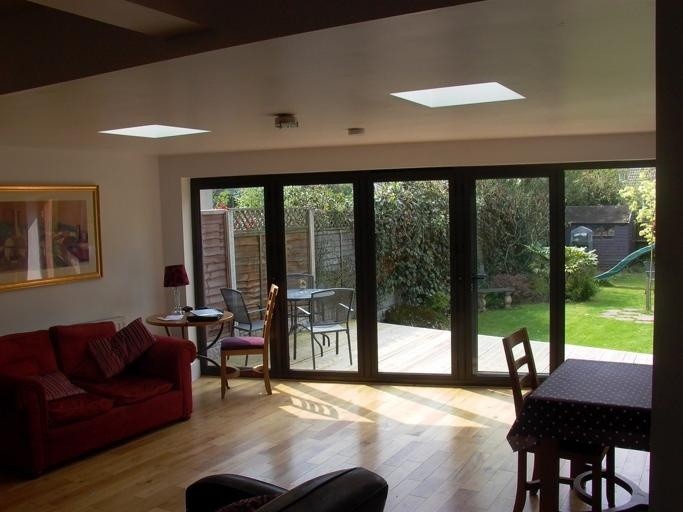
[
  {"xmin": 86, "ymin": 316, "xmax": 156, "ymax": 380},
  {"xmin": 27, "ymin": 369, "xmax": 88, "ymax": 401}
]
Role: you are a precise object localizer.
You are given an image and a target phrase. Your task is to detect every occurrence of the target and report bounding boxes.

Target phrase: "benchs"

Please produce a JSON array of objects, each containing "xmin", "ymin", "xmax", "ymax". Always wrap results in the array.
[{"xmin": 478, "ymin": 287, "xmax": 515, "ymax": 312}]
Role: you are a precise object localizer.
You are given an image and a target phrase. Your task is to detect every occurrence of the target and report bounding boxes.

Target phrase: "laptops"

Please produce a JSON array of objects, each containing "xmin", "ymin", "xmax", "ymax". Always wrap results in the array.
[{"xmin": 187, "ymin": 308, "xmax": 223, "ymax": 321}]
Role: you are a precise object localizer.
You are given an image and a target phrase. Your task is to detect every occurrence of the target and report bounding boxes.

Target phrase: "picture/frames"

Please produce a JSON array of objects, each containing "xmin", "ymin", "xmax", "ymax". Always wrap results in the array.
[{"xmin": 0, "ymin": 184, "xmax": 103, "ymax": 293}]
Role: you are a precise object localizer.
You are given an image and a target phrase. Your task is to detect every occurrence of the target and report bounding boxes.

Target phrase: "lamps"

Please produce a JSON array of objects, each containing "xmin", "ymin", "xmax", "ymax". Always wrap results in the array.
[{"xmin": 164, "ymin": 264, "xmax": 190, "ymax": 314}]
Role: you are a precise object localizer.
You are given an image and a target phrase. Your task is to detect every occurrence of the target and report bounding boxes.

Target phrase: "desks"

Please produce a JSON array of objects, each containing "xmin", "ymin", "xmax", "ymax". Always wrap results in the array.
[
  {"xmin": 524, "ymin": 358, "xmax": 653, "ymax": 511},
  {"xmin": 145, "ymin": 311, "xmax": 233, "ymax": 389}
]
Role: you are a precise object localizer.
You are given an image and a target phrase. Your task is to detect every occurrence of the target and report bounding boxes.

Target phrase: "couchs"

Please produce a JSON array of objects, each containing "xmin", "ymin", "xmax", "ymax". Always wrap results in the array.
[{"xmin": 0, "ymin": 321, "xmax": 196, "ymax": 477}]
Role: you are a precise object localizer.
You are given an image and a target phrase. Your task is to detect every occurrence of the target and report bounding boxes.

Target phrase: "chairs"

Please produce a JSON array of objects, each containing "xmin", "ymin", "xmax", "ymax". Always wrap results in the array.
[
  {"xmin": 501, "ymin": 326, "xmax": 615, "ymax": 511},
  {"xmin": 220, "ymin": 284, "xmax": 279, "ymax": 400},
  {"xmin": 184, "ymin": 467, "xmax": 388, "ymax": 512},
  {"xmin": 220, "ymin": 274, "xmax": 353, "ymax": 370}
]
